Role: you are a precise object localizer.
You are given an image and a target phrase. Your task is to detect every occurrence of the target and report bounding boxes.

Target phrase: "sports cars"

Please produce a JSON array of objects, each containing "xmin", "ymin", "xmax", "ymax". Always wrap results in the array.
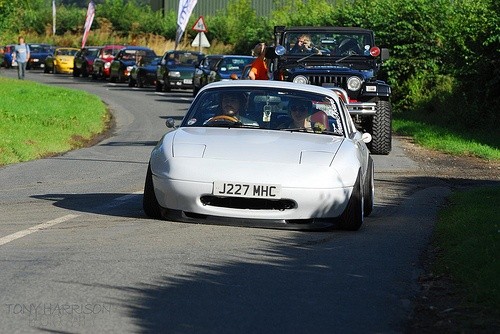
[
  {"xmin": 44, "ymin": 47, "xmax": 81, "ymax": 74},
  {"xmin": 143, "ymin": 80, "xmax": 375, "ymax": 232}
]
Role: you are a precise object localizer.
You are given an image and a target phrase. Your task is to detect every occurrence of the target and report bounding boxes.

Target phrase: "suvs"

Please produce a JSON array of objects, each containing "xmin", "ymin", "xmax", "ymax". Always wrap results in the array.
[{"xmin": 263, "ymin": 25, "xmax": 391, "ymax": 155}]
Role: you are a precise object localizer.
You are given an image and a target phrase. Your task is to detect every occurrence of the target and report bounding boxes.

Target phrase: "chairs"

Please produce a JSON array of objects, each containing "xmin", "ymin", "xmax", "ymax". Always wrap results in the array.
[
  {"xmin": 312, "ymin": 108, "xmax": 329, "ymax": 130},
  {"xmin": 338, "ymin": 37, "xmax": 363, "ymax": 56}
]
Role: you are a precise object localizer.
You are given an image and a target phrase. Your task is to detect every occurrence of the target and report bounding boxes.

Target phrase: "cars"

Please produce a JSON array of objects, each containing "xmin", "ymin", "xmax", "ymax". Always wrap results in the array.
[
  {"xmin": 72, "ymin": 45, "xmax": 205, "ymax": 92},
  {"xmin": 0, "ymin": 42, "xmax": 57, "ymax": 70}
]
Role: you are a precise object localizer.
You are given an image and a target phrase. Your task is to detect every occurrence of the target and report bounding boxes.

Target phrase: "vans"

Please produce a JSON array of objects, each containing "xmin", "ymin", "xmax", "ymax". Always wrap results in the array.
[{"xmin": 192, "ymin": 53, "xmax": 260, "ymax": 96}]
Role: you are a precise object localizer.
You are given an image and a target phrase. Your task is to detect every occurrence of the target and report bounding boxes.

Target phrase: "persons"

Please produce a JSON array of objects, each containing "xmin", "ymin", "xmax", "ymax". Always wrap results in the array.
[
  {"xmin": 275, "ymin": 98, "xmax": 326, "ymax": 133},
  {"xmin": 199, "ymin": 91, "xmax": 259, "ymax": 128},
  {"xmin": 287, "ymin": 33, "xmax": 321, "ymax": 56},
  {"xmin": 13, "ymin": 36, "xmax": 31, "ymax": 80}
]
